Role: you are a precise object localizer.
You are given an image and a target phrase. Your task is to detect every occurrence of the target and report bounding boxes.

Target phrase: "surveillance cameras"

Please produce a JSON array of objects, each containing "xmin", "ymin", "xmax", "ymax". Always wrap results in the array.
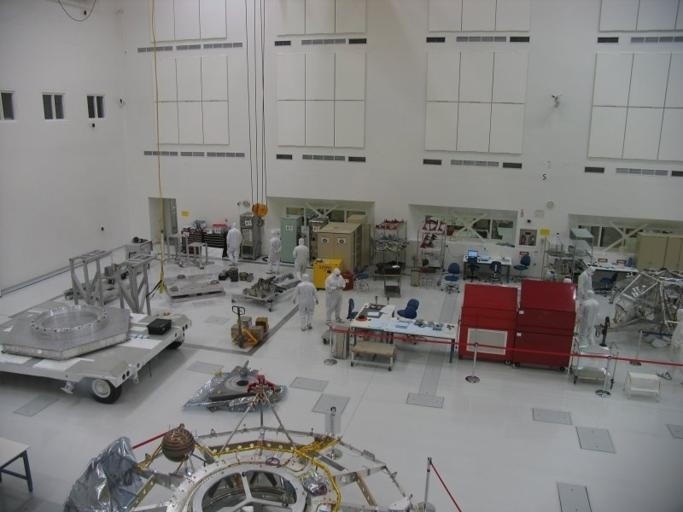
[{"xmin": 551, "ymin": 91, "xmax": 562, "ymax": 99}]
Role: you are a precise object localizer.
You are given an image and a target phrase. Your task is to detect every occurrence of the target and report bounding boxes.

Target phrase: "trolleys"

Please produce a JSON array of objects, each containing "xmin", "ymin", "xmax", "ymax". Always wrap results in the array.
[{"xmin": 570, "ymin": 339, "xmax": 619, "ymax": 385}]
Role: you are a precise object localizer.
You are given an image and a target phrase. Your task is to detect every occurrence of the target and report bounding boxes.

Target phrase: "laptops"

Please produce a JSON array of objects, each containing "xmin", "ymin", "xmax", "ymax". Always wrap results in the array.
[{"xmin": 467, "ymin": 249, "xmax": 478, "ymax": 261}]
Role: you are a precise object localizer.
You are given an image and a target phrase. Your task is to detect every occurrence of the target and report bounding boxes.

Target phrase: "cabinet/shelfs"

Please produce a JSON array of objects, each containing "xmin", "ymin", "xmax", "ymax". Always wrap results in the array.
[{"xmin": 635, "ymin": 232, "xmax": 682, "ymax": 272}]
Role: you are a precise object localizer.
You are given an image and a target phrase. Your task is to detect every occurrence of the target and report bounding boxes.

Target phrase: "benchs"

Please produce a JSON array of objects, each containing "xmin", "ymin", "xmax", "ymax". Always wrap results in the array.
[{"xmin": 351, "ymin": 342, "xmax": 398, "ymax": 370}]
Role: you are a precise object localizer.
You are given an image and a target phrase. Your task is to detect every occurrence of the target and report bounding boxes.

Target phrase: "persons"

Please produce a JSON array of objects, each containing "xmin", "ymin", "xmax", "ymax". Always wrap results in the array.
[
  {"xmin": 226, "ymin": 223, "xmax": 243, "ymax": 266},
  {"xmin": 293, "ymin": 274, "xmax": 319, "ymax": 331},
  {"xmin": 578, "ymin": 266, "xmax": 596, "ymax": 298},
  {"xmin": 325, "ymin": 268, "xmax": 346, "ymax": 326},
  {"xmin": 293, "ymin": 238, "xmax": 308, "ymax": 280},
  {"xmin": 577, "ymin": 290, "xmax": 599, "ymax": 347},
  {"xmin": 265, "ymin": 230, "xmax": 281, "ymax": 275}
]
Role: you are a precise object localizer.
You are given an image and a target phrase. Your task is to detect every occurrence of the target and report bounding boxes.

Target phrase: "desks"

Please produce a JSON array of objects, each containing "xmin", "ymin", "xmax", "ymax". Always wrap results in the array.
[
  {"xmin": 587, "ymin": 265, "xmax": 639, "ymax": 278},
  {"xmin": 350, "ymin": 303, "xmax": 459, "ymax": 363}
]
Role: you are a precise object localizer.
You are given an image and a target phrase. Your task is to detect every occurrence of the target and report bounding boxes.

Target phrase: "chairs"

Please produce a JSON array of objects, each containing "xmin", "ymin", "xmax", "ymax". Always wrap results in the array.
[
  {"xmin": 462, "ymin": 257, "xmax": 512, "ymax": 283},
  {"xmin": 465, "ymin": 258, "xmax": 502, "ymax": 284},
  {"xmin": 441, "ymin": 263, "xmax": 461, "ymax": 294},
  {"xmin": 345, "ymin": 298, "xmax": 357, "ymax": 321},
  {"xmin": 514, "ymin": 254, "xmax": 530, "ymax": 282}
]
